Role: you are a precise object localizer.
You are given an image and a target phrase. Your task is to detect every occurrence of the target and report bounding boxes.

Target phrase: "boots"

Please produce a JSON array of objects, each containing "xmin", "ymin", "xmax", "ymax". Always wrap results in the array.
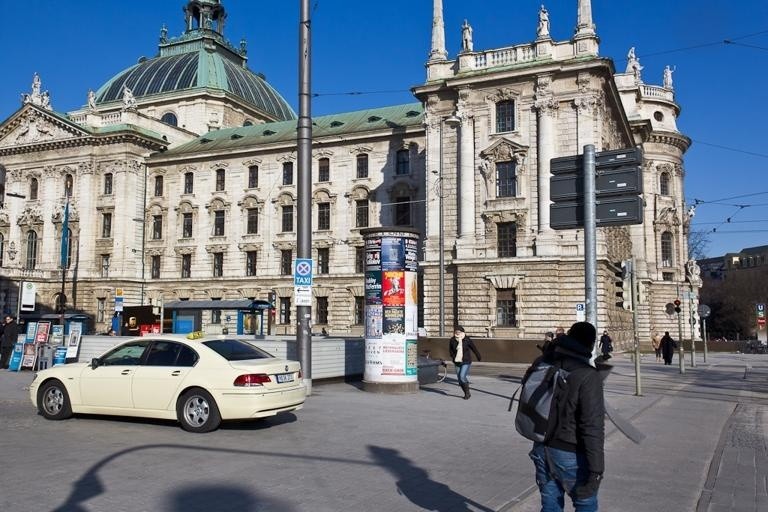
[{"xmin": 461, "ymin": 382, "xmax": 471, "ymax": 399}]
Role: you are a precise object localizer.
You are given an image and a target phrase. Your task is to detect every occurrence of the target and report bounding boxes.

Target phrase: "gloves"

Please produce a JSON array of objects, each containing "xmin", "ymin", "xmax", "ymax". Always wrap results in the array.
[{"xmin": 577, "ymin": 473, "xmax": 603, "ymax": 499}]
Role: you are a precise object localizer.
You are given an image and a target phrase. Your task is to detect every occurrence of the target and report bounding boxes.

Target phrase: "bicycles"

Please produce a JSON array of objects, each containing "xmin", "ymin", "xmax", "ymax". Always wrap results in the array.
[{"xmin": 420, "ymin": 348, "xmax": 447, "ymax": 383}]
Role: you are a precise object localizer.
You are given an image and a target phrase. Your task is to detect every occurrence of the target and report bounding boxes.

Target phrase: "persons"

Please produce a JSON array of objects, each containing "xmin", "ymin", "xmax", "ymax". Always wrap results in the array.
[
  {"xmin": 122, "ymin": 85, "xmax": 137, "ymax": 105},
  {"xmin": 599, "ymin": 331, "xmax": 612, "ymax": 356},
  {"xmin": 0, "ymin": 315, "xmax": 18, "ymax": 369},
  {"xmin": 31, "ymin": 72, "xmax": 41, "ymax": 103},
  {"xmin": 536, "ymin": 4, "xmax": 550, "ymax": 36},
  {"xmin": 663, "ymin": 65, "xmax": 676, "ymax": 90},
  {"xmin": 461, "ymin": 19, "xmax": 474, "ymax": 52},
  {"xmin": 528, "ymin": 322, "xmax": 604, "ymax": 512},
  {"xmin": 625, "ymin": 47, "xmax": 644, "ymax": 72},
  {"xmin": 21, "ymin": 93, "xmax": 31, "ymax": 104},
  {"xmin": 547, "ymin": 327, "xmax": 566, "ymax": 352},
  {"xmin": 42, "ymin": 90, "xmax": 53, "ymax": 111},
  {"xmin": 536, "ymin": 332, "xmax": 553, "ymax": 355},
  {"xmin": 652, "ymin": 332, "xmax": 662, "ymax": 362},
  {"xmin": 658, "ymin": 332, "xmax": 677, "ymax": 365},
  {"xmin": 87, "ymin": 88, "xmax": 96, "ymax": 109},
  {"xmin": 449, "ymin": 326, "xmax": 482, "ymax": 400}
]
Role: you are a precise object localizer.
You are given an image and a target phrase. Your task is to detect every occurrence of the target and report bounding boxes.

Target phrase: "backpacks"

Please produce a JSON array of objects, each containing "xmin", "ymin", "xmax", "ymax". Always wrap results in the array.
[{"xmin": 508, "ymin": 356, "xmax": 594, "ymax": 442}]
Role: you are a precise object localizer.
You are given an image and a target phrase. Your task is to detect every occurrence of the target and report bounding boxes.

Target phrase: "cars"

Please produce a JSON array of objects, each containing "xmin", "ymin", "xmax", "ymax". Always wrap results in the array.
[{"xmin": 30, "ymin": 329, "xmax": 307, "ymax": 434}]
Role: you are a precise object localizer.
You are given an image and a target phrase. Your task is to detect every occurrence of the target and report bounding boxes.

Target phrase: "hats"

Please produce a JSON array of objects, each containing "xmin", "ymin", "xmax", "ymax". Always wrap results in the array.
[{"xmin": 568, "ymin": 321, "xmax": 596, "ymax": 352}]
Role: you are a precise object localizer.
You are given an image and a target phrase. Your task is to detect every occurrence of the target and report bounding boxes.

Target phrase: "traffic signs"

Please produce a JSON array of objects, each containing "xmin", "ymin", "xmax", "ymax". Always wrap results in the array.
[{"xmin": 756, "ymin": 304, "xmax": 765, "ymax": 317}]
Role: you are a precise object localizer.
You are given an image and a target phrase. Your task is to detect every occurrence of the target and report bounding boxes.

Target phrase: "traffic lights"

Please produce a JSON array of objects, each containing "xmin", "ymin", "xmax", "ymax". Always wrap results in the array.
[
  {"xmin": 614, "ymin": 260, "xmax": 633, "ymax": 311},
  {"xmin": 675, "ymin": 300, "xmax": 681, "ymax": 313}
]
